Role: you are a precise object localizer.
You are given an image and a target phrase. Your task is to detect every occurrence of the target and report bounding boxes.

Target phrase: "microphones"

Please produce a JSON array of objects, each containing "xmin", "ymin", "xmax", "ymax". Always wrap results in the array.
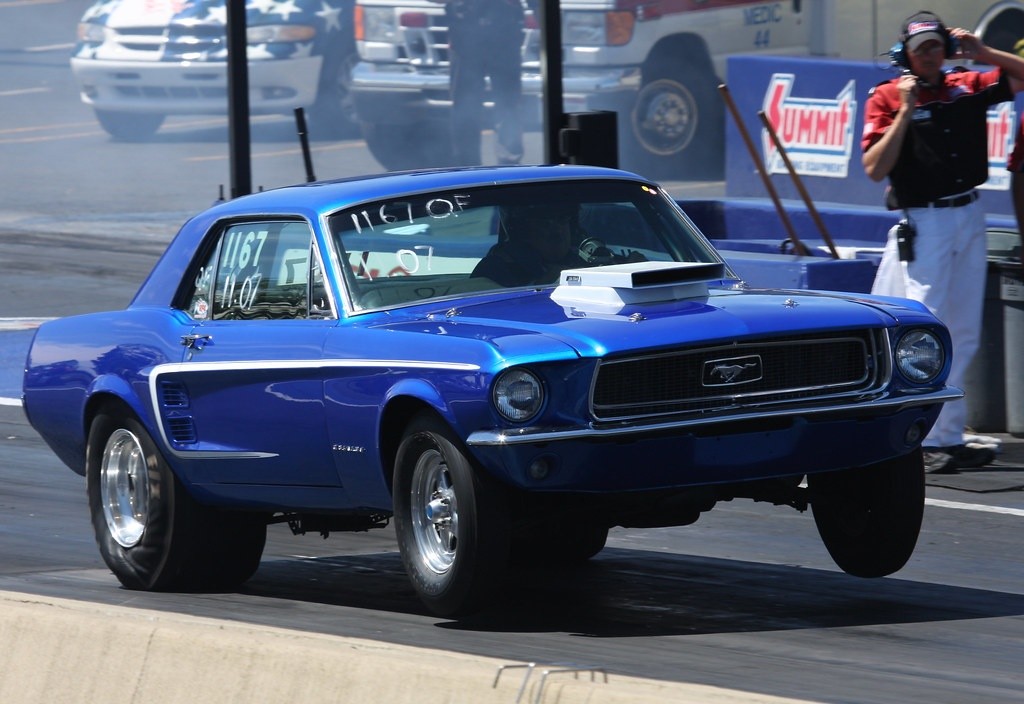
[{"xmin": 890, "ymin": 61, "xmax": 927, "ymax": 89}]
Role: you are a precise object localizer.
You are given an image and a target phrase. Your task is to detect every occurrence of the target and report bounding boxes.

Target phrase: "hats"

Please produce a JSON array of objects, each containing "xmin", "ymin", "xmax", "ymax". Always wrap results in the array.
[{"xmin": 902, "ymin": 10, "xmax": 946, "ymax": 51}]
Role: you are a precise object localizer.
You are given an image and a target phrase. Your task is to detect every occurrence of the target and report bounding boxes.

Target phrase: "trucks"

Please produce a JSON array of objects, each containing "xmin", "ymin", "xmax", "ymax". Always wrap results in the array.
[{"xmin": 348, "ymin": 0, "xmax": 1024, "ymax": 184}]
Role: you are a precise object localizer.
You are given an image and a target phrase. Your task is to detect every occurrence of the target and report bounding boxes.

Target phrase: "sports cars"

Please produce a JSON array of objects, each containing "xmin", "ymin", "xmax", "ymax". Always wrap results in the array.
[{"xmin": 23, "ymin": 162, "xmax": 966, "ymax": 625}]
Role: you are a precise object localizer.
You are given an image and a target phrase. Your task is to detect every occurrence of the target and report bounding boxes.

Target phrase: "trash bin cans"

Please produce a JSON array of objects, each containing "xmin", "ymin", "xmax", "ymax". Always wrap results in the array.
[{"xmin": 968, "ymin": 260, "xmax": 1024, "ymax": 436}]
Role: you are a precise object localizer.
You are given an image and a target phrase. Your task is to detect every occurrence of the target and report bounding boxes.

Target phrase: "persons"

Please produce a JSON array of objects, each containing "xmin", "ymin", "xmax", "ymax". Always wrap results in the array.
[
  {"xmin": 467, "ymin": 203, "xmax": 646, "ymax": 285},
  {"xmin": 863, "ymin": 11, "xmax": 1023, "ymax": 474},
  {"xmin": 445, "ymin": 0, "xmax": 539, "ymax": 169}
]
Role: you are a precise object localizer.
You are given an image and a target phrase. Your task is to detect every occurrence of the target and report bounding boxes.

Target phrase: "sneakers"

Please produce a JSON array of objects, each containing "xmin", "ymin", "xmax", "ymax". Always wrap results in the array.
[
  {"xmin": 920, "ymin": 445, "xmax": 955, "ymax": 473},
  {"xmin": 944, "ymin": 442, "xmax": 998, "ymax": 468}
]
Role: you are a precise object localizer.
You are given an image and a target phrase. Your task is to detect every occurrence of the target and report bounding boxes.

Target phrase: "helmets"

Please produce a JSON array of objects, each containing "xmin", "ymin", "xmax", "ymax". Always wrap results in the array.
[{"xmin": 493, "ymin": 201, "xmax": 582, "ymax": 265}]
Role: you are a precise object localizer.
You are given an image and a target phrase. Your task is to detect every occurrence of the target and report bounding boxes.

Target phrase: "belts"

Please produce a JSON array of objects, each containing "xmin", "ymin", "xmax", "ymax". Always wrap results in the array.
[{"xmin": 919, "ymin": 192, "xmax": 979, "ymax": 207}]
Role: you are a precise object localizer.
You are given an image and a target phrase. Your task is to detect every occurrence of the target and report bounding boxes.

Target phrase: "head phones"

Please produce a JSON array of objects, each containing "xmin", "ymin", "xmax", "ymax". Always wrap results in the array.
[{"xmin": 889, "ymin": 10, "xmax": 960, "ymax": 71}]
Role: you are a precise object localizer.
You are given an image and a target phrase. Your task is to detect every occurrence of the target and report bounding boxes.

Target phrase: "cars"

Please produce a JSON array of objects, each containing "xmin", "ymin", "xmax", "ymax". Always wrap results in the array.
[{"xmin": 67, "ymin": 1, "xmax": 357, "ymax": 146}]
